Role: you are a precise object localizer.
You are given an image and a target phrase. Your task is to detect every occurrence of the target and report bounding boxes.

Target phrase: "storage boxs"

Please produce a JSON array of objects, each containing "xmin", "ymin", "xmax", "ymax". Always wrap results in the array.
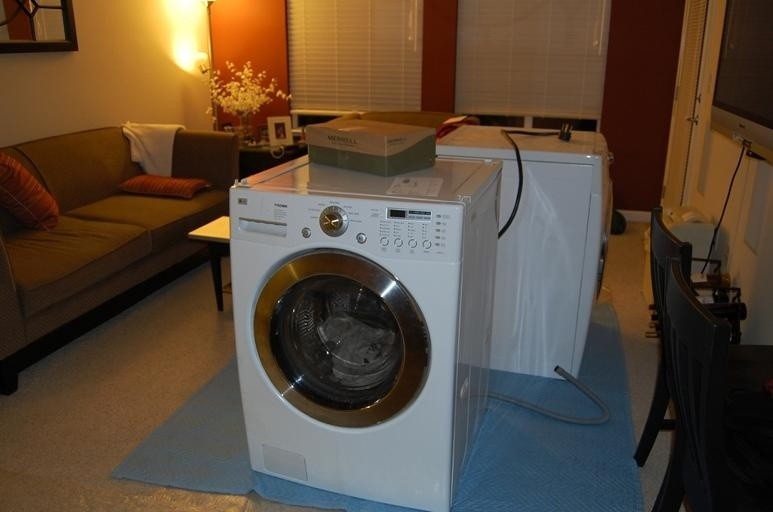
[{"xmin": 305, "ymin": 115, "xmax": 436, "ymax": 176}]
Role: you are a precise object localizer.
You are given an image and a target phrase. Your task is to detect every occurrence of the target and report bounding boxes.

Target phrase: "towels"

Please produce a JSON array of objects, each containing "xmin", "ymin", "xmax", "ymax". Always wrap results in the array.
[{"xmin": 121, "ymin": 118, "xmax": 185, "ymax": 177}]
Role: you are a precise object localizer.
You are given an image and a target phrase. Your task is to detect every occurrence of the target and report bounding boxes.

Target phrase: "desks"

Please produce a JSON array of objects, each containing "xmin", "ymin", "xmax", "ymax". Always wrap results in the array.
[
  {"xmin": 238, "ymin": 138, "xmax": 307, "ymax": 180},
  {"xmin": 189, "ymin": 212, "xmax": 233, "ymax": 314}
]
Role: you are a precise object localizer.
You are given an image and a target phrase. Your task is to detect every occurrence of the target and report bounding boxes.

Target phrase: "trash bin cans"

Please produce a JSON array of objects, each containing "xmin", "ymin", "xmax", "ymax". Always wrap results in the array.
[{"xmin": 642, "ymin": 206, "xmax": 720, "ymax": 315}]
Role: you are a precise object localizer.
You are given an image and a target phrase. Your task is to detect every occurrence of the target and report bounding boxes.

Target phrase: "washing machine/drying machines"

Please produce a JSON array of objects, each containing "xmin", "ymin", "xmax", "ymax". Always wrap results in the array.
[
  {"xmin": 435, "ymin": 125, "xmax": 612, "ymax": 382},
  {"xmin": 229, "ymin": 154, "xmax": 502, "ymax": 511}
]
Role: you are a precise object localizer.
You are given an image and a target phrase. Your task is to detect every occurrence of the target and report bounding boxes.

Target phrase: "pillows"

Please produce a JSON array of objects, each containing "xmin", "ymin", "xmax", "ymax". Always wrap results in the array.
[
  {"xmin": 0, "ymin": 154, "xmax": 56, "ymax": 229},
  {"xmin": 118, "ymin": 172, "xmax": 209, "ymax": 199}
]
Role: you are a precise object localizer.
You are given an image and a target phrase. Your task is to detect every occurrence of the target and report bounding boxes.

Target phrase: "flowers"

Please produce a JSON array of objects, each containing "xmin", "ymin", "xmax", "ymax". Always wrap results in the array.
[{"xmin": 201, "ymin": 59, "xmax": 292, "ymax": 117}]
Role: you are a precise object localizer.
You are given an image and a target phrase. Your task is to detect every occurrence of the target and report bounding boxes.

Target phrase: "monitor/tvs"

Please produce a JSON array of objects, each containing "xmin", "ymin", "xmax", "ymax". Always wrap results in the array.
[{"xmin": 711, "ymin": 0, "xmax": 773, "ymax": 165}]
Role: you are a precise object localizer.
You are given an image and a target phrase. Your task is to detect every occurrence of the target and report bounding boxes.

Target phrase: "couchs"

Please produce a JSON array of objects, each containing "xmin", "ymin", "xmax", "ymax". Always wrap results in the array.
[{"xmin": 0, "ymin": 119, "xmax": 241, "ymax": 392}]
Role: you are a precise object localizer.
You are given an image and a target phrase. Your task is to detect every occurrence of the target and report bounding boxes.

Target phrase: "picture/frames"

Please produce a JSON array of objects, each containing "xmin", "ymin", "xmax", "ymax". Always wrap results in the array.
[
  {"xmin": 0, "ymin": 0, "xmax": 80, "ymax": 53},
  {"xmin": 266, "ymin": 116, "xmax": 294, "ymax": 145}
]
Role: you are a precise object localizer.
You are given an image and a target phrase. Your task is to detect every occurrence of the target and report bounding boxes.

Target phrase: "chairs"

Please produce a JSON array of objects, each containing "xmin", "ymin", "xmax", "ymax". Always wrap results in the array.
[
  {"xmin": 633, "ymin": 204, "xmax": 773, "ymax": 471},
  {"xmin": 644, "ymin": 257, "xmax": 773, "ymax": 511}
]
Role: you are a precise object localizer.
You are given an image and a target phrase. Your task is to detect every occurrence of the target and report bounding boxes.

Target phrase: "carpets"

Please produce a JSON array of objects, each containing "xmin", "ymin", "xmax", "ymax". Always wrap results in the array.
[{"xmin": 107, "ymin": 297, "xmax": 649, "ymax": 511}]
